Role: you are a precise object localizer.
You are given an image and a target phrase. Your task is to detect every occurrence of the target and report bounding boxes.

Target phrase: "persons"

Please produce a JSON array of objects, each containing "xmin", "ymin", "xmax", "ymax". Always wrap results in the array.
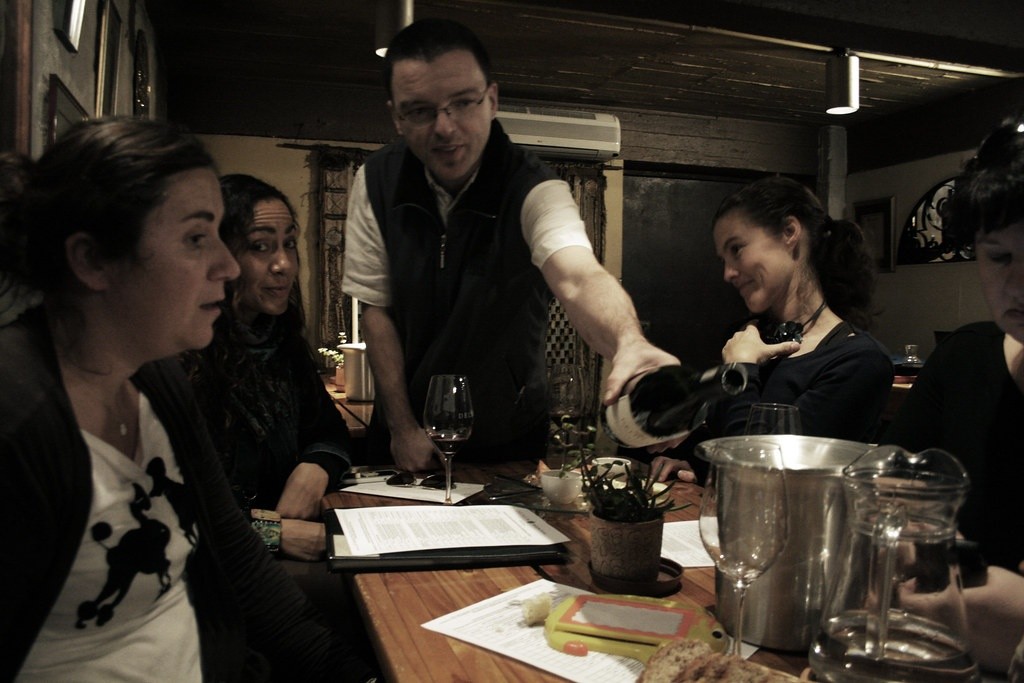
[
  {"xmin": 0, "ymin": 114, "xmax": 240, "ymax": 683},
  {"xmin": 339, "ymin": 19, "xmax": 680, "ymax": 478},
  {"xmin": 890, "ymin": 124, "xmax": 1024, "ymax": 683},
  {"xmin": 150, "ymin": 174, "xmax": 372, "ymax": 683},
  {"xmin": 693, "ymin": 173, "xmax": 896, "ymax": 449}
]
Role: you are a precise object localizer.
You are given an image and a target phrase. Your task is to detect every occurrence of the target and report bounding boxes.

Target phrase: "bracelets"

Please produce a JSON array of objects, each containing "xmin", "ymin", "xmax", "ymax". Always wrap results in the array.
[{"xmin": 250, "ymin": 508, "xmax": 282, "ymax": 553}]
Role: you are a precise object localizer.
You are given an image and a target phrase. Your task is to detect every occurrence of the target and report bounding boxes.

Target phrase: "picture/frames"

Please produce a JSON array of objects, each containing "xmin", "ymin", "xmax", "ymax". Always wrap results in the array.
[
  {"xmin": 853, "ymin": 194, "xmax": 896, "ymax": 275},
  {"xmin": 45, "ymin": 72, "xmax": 90, "ymax": 148}
]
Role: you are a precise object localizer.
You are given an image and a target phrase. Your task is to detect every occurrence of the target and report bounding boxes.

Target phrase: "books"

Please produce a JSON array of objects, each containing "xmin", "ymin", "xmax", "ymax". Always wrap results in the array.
[{"xmin": 322, "ymin": 506, "xmax": 569, "ymax": 574}]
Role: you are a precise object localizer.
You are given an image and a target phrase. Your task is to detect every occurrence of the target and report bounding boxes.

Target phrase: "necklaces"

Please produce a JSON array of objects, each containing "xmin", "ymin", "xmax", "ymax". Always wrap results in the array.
[
  {"xmin": 770, "ymin": 301, "xmax": 826, "ymax": 347},
  {"xmin": 63, "ymin": 374, "xmax": 129, "ymax": 438}
]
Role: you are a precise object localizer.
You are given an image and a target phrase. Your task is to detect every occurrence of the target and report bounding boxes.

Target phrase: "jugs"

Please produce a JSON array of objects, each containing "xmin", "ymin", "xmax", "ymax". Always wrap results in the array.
[{"xmin": 807, "ymin": 443, "xmax": 980, "ymax": 683}]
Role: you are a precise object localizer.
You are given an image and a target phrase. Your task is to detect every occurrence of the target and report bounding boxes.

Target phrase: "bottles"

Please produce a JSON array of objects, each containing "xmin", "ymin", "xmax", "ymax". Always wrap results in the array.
[{"xmin": 600, "ymin": 363, "xmax": 748, "ymax": 449}]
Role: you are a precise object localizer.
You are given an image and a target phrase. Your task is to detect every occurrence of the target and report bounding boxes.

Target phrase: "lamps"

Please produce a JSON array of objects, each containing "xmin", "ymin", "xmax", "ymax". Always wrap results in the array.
[
  {"xmin": 373, "ymin": 0, "xmax": 415, "ymax": 59},
  {"xmin": 822, "ymin": 46, "xmax": 861, "ymax": 116}
]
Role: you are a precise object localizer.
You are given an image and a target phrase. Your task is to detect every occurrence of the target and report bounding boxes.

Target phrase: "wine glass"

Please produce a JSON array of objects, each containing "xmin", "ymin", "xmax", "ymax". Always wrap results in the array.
[
  {"xmin": 698, "ymin": 438, "xmax": 792, "ymax": 657},
  {"xmin": 423, "ymin": 374, "xmax": 474, "ymax": 506}
]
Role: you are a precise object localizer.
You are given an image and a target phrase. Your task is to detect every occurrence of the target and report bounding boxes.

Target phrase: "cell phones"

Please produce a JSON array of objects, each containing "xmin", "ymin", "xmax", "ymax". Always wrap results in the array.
[{"xmin": 340, "ymin": 470, "xmax": 399, "ymax": 484}]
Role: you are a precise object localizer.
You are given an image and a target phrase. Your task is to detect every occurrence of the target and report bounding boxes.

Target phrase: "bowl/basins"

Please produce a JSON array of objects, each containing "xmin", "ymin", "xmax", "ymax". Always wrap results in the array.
[{"xmin": 591, "ymin": 457, "xmax": 631, "ymax": 481}]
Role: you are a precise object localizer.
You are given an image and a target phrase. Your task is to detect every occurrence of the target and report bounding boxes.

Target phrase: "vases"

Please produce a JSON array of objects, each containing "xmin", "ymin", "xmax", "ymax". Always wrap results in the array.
[{"xmin": 319, "ymin": 331, "xmax": 347, "ymax": 393}]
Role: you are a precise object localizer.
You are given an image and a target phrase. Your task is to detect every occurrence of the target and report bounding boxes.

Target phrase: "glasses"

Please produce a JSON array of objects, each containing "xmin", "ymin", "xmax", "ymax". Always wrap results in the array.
[
  {"xmin": 966, "ymin": 119, "xmax": 1024, "ymax": 169},
  {"xmin": 387, "ymin": 471, "xmax": 455, "ymax": 491},
  {"xmin": 391, "ymin": 82, "xmax": 489, "ymax": 130}
]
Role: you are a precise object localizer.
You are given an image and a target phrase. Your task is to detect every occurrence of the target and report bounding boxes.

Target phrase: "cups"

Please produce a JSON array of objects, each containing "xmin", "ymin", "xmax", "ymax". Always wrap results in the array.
[
  {"xmin": 744, "ymin": 402, "xmax": 804, "ymax": 436},
  {"xmin": 905, "ymin": 345, "xmax": 919, "ymax": 364}
]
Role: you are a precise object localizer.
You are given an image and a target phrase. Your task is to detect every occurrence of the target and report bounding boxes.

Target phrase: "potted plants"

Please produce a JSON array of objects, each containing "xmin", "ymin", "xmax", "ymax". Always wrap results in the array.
[
  {"xmin": 575, "ymin": 460, "xmax": 695, "ymax": 597},
  {"xmin": 539, "ymin": 414, "xmax": 597, "ymax": 506}
]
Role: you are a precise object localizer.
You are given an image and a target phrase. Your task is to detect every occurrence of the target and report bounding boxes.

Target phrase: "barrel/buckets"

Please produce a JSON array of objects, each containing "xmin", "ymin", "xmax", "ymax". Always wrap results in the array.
[{"xmin": 695, "ymin": 433, "xmax": 878, "ymax": 652}]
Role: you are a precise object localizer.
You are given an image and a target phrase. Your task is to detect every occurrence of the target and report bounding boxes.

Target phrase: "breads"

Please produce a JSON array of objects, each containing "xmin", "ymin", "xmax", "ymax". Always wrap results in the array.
[{"xmin": 636, "ymin": 638, "xmax": 772, "ymax": 683}]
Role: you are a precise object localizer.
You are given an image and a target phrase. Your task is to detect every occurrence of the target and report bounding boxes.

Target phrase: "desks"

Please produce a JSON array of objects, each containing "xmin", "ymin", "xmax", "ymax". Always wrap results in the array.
[
  {"xmin": 322, "ymin": 459, "xmax": 943, "ymax": 683},
  {"xmin": 321, "ymin": 373, "xmax": 376, "ymax": 440}
]
